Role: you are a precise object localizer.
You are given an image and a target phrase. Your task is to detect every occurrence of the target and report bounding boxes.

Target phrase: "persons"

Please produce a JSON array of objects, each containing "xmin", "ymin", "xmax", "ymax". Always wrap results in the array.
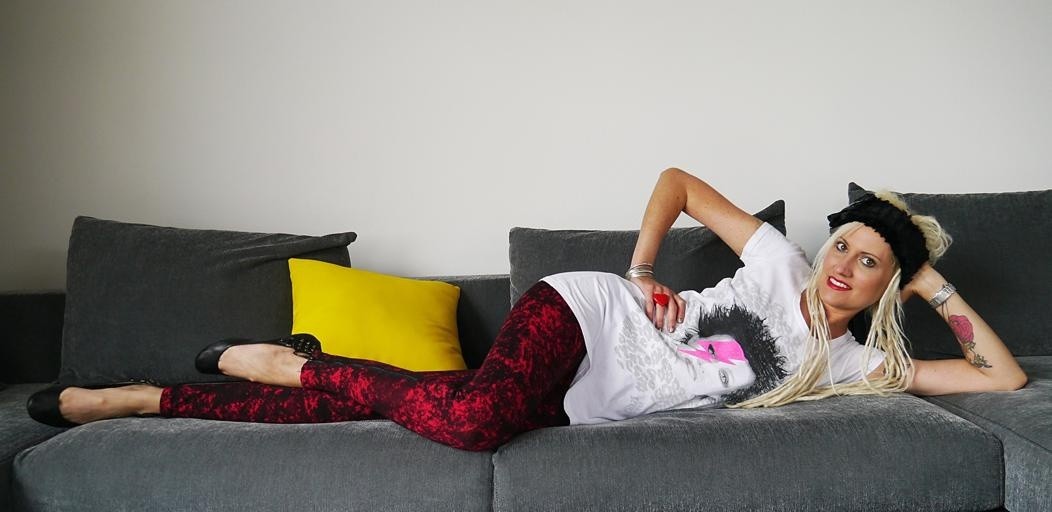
[
  {"xmin": 26, "ymin": 167, "xmax": 1029, "ymax": 450},
  {"xmin": 671, "ymin": 304, "xmax": 791, "ymax": 406}
]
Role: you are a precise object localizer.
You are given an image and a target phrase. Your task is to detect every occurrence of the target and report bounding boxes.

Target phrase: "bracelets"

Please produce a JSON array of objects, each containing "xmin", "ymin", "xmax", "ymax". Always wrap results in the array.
[
  {"xmin": 928, "ymin": 280, "xmax": 955, "ymax": 309},
  {"xmin": 624, "ymin": 261, "xmax": 656, "ymax": 279}
]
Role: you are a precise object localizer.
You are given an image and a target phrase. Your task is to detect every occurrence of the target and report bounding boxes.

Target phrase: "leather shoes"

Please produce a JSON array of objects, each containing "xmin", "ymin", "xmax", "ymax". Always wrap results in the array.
[
  {"xmin": 195, "ymin": 333, "xmax": 322, "ymax": 381},
  {"xmin": 24, "ymin": 381, "xmax": 157, "ymax": 427}
]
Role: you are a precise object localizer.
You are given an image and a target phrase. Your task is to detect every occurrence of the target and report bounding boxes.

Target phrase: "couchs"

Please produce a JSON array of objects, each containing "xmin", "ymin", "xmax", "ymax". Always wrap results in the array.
[{"xmin": 1, "ymin": 182, "xmax": 1052, "ymax": 511}]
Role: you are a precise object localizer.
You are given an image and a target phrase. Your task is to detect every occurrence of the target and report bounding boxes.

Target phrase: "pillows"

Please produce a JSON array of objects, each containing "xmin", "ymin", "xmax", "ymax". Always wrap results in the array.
[{"xmin": 284, "ymin": 256, "xmax": 467, "ymax": 374}]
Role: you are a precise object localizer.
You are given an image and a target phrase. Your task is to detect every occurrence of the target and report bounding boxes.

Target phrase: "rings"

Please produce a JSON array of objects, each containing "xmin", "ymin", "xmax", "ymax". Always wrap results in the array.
[{"xmin": 652, "ymin": 292, "xmax": 671, "ymax": 308}]
